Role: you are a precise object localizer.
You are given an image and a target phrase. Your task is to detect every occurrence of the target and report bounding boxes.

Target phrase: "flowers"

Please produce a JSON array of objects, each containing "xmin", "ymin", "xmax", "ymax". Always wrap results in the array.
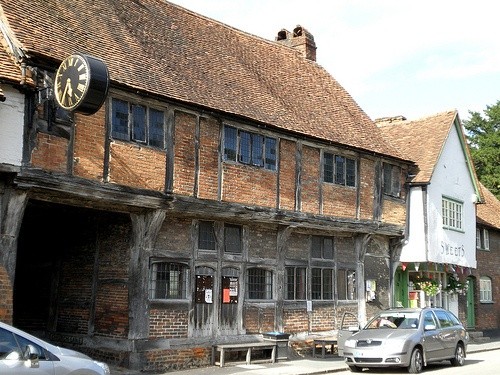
[
  {"xmin": 415, "ymin": 277, "xmax": 442, "ymax": 295},
  {"xmin": 446, "ymin": 275, "xmax": 469, "ymax": 296}
]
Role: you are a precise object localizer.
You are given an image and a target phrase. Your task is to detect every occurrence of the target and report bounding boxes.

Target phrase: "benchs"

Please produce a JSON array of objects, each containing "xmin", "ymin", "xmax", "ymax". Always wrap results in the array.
[
  {"xmin": 313, "ymin": 339, "xmax": 338, "ymax": 359},
  {"xmin": 212, "ymin": 342, "xmax": 277, "ymax": 367}
]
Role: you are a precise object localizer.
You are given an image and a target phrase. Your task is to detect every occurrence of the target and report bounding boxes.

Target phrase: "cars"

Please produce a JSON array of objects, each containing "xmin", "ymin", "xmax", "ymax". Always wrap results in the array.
[
  {"xmin": 0, "ymin": 321, "xmax": 110, "ymax": 375},
  {"xmin": 336, "ymin": 305, "xmax": 470, "ymax": 374}
]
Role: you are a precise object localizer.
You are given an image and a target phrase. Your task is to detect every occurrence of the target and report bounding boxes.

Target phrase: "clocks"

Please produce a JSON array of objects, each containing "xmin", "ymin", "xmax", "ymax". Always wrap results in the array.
[{"xmin": 54, "ymin": 54, "xmax": 110, "ymax": 115}]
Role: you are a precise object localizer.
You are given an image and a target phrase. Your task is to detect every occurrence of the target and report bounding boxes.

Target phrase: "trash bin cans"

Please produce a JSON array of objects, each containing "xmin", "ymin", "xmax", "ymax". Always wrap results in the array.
[{"xmin": 263, "ymin": 332, "xmax": 290, "ymax": 362}]
